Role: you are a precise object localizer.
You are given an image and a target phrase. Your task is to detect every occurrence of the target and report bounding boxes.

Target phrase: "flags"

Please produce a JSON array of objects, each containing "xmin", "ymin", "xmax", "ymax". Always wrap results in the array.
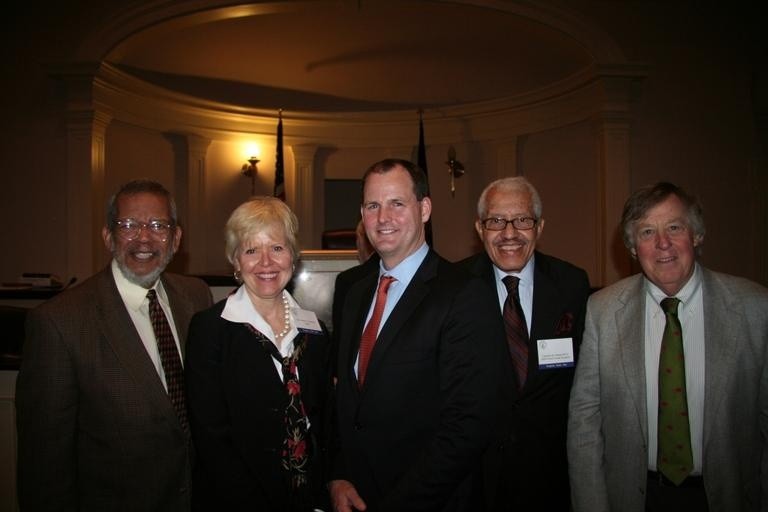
[
  {"xmin": 274, "ymin": 119, "xmax": 287, "ymax": 202},
  {"xmin": 417, "ymin": 116, "xmax": 435, "ymax": 250}
]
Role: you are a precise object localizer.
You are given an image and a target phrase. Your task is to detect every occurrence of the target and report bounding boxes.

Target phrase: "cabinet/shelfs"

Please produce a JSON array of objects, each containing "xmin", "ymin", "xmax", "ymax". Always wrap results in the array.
[{"xmin": 0, "ymin": 299, "xmax": 45, "ymax": 512}]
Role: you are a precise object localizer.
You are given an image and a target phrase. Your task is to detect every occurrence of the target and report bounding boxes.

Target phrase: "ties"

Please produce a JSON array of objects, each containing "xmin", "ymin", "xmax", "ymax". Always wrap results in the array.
[
  {"xmin": 656, "ymin": 299, "xmax": 695, "ymax": 487},
  {"xmin": 502, "ymin": 276, "xmax": 529, "ymax": 394},
  {"xmin": 359, "ymin": 276, "xmax": 398, "ymax": 394},
  {"xmin": 145, "ymin": 289, "xmax": 190, "ymax": 439}
]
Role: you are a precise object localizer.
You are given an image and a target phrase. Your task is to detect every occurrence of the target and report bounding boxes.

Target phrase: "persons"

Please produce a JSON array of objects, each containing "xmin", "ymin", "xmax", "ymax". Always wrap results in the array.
[
  {"xmin": 564, "ymin": 181, "xmax": 768, "ymax": 512},
  {"xmin": 356, "ymin": 218, "xmax": 375, "ymax": 265},
  {"xmin": 185, "ymin": 195, "xmax": 336, "ymax": 510},
  {"xmin": 333, "ymin": 157, "xmax": 507, "ymax": 510},
  {"xmin": 451, "ymin": 173, "xmax": 589, "ymax": 512},
  {"xmin": 15, "ymin": 177, "xmax": 215, "ymax": 512}
]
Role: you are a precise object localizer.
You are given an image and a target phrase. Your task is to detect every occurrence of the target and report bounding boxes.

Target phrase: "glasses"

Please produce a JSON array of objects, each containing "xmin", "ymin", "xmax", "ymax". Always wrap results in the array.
[
  {"xmin": 112, "ymin": 218, "xmax": 174, "ymax": 241},
  {"xmin": 482, "ymin": 216, "xmax": 538, "ymax": 231}
]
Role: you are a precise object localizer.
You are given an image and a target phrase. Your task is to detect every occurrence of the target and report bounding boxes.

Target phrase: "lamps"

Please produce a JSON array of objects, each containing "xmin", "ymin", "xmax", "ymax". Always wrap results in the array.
[
  {"xmin": 242, "ymin": 144, "xmax": 260, "ymax": 196},
  {"xmin": 444, "ymin": 145, "xmax": 464, "ymax": 198}
]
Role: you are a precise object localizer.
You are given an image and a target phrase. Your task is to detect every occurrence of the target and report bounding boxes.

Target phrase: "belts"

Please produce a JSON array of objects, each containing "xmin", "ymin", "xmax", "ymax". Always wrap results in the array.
[{"xmin": 648, "ymin": 475, "xmax": 703, "ymax": 486}]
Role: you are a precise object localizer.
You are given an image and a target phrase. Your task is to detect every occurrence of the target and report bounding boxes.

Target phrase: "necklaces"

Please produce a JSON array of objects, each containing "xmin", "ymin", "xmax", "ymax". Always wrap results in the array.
[{"xmin": 247, "ymin": 290, "xmax": 290, "ymax": 339}]
